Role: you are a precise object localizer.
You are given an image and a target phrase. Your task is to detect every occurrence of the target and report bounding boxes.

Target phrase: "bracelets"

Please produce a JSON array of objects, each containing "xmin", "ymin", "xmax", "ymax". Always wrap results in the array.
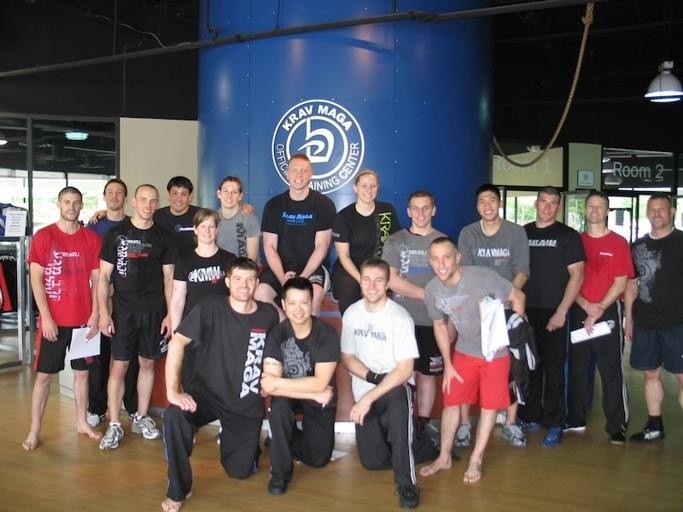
[{"xmin": 366, "ymin": 369, "xmax": 388, "ymax": 385}]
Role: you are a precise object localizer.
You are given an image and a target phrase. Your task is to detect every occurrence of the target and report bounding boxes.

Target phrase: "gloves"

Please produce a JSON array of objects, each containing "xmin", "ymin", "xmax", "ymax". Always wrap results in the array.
[{"xmin": 366, "ymin": 370, "xmax": 388, "ymax": 384}]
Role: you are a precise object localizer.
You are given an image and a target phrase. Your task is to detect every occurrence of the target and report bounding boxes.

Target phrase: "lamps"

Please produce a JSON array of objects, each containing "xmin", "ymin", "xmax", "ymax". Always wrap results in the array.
[
  {"xmin": 643, "ymin": 60, "xmax": 682, "ymax": 107},
  {"xmin": 0, "ymin": 132, "xmax": 7, "ymax": 148},
  {"xmin": 64, "ymin": 121, "xmax": 88, "ymax": 142}
]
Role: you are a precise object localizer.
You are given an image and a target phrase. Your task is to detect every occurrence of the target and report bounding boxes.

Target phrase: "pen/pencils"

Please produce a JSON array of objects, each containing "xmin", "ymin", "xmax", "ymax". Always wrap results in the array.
[
  {"xmin": 108, "ymin": 325, "xmax": 112, "ymax": 334},
  {"xmin": 581, "ymin": 321, "xmax": 596, "ymax": 327}
]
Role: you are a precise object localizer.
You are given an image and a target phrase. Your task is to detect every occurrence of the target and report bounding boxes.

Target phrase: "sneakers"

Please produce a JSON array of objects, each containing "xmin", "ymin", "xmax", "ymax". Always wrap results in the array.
[
  {"xmin": 501, "ymin": 425, "xmax": 527, "ymax": 448},
  {"xmin": 610, "ymin": 423, "xmax": 628, "ymax": 443},
  {"xmin": 630, "ymin": 425, "xmax": 665, "ymax": 442},
  {"xmin": 454, "ymin": 424, "xmax": 473, "ymax": 449},
  {"xmin": 543, "ymin": 424, "xmax": 563, "ymax": 446},
  {"xmin": 394, "ymin": 484, "xmax": 420, "ymax": 509},
  {"xmin": 87, "ymin": 411, "xmax": 106, "ymax": 428},
  {"xmin": 423, "ymin": 423, "xmax": 460, "ymax": 459},
  {"xmin": 561, "ymin": 420, "xmax": 586, "ymax": 432},
  {"xmin": 99, "ymin": 424, "xmax": 125, "ymax": 451},
  {"xmin": 515, "ymin": 417, "xmax": 541, "ymax": 432},
  {"xmin": 268, "ymin": 478, "xmax": 286, "ymax": 494},
  {"xmin": 130, "ymin": 411, "xmax": 161, "ymax": 440}
]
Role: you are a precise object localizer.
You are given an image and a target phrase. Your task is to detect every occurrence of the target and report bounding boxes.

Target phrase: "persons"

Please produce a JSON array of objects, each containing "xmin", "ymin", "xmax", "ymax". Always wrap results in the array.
[
  {"xmin": 22, "ymin": 186, "xmax": 104, "ymax": 453},
  {"xmin": 259, "ymin": 276, "xmax": 340, "ymax": 496},
  {"xmin": 96, "ymin": 183, "xmax": 178, "ymax": 452},
  {"xmin": 340, "ymin": 258, "xmax": 462, "ymax": 508},
  {"xmin": 83, "ymin": 179, "xmax": 138, "ymax": 428},
  {"xmin": 169, "ymin": 207, "xmax": 237, "ymax": 448},
  {"xmin": 89, "ymin": 175, "xmax": 261, "ymax": 263},
  {"xmin": 160, "ymin": 256, "xmax": 280, "ymax": 512},
  {"xmin": 328, "ymin": 167, "xmax": 402, "ymax": 316},
  {"xmin": 252, "ymin": 153, "xmax": 337, "ymax": 449}
]
